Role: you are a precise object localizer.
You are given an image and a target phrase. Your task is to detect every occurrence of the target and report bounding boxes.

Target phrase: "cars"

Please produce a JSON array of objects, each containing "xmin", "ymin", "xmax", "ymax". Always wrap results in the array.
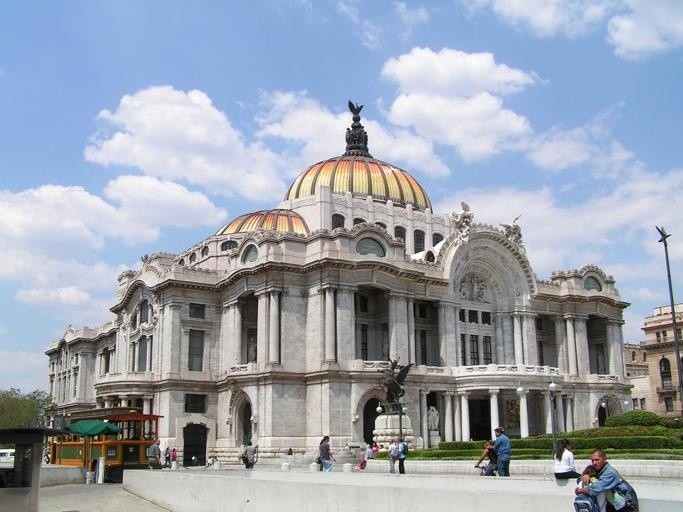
[{"xmin": 0, "ymin": 449, "xmax": 15, "ymax": 468}]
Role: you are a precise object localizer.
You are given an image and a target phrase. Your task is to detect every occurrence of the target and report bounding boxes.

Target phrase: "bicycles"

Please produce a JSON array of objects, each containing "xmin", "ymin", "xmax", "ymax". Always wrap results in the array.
[
  {"xmin": 43, "ymin": 448, "xmax": 50, "ymax": 464},
  {"xmin": 475, "ymin": 463, "xmax": 487, "ymax": 476}
]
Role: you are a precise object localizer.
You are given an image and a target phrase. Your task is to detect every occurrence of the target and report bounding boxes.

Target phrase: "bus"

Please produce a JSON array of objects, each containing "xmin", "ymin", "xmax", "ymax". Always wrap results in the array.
[{"xmin": 50, "ymin": 406, "xmax": 164, "ymax": 482}]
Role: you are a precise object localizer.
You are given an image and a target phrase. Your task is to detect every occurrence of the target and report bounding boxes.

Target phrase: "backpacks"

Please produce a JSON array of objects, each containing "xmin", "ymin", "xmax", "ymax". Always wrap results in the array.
[
  {"xmin": 242, "ymin": 455, "xmax": 249, "ymax": 464},
  {"xmin": 573, "ymin": 493, "xmax": 600, "ymax": 512},
  {"xmin": 400, "ymin": 444, "xmax": 408, "ymax": 455}
]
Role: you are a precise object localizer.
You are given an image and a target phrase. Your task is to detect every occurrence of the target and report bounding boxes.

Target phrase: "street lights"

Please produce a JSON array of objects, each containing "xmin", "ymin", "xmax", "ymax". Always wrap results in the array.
[
  {"xmin": 601, "ymin": 398, "xmax": 608, "ymax": 418},
  {"xmin": 376, "ymin": 401, "xmax": 408, "ymax": 442},
  {"xmin": 548, "ymin": 383, "xmax": 562, "ymax": 457}
]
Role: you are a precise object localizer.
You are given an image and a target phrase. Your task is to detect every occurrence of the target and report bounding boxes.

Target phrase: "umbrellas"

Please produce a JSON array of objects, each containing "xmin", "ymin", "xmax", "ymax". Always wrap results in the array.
[{"xmin": 62, "ymin": 419, "xmax": 121, "ymax": 469}]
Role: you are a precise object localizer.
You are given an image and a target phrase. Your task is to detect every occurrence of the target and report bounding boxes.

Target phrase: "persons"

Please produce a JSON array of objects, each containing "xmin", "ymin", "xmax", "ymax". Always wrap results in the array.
[
  {"xmin": 551, "ymin": 439, "xmax": 581, "ymax": 479},
  {"xmin": 169, "ymin": 447, "xmax": 177, "ymax": 463},
  {"xmin": 147, "ymin": 437, "xmax": 162, "ymax": 469},
  {"xmin": 431, "ymin": 407, "xmax": 439, "ymax": 430},
  {"xmin": 474, "ymin": 441, "xmax": 498, "ymax": 476},
  {"xmin": 375, "ymin": 355, "xmax": 400, "ymax": 379},
  {"xmin": 367, "ymin": 438, "xmax": 386, "ymax": 460},
  {"xmin": 288, "ymin": 446, "xmax": 293, "ymax": 456},
  {"xmin": 388, "ymin": 438, "xmax": 398, "ymax": 473},
  {"xmin": 486, "ymin": 426, "xmax": 511, "ymax": 477},
  {"xmin": 320, "ymin": 435, "xmax": 334, "ymax": 471},
  {"xmin": 345, "ymin": 125, "xmax": 368, "ymax": 149},
  {"xmin": 427, "ymin": 406, "xmax": 433, "ymax": 431},
  {"xmin": 44, "ymin": 444, "xmax": 50, "ymax": 451},
  {"xmin": 573, "ymin": 447, "xmax": 638, "ymax": 512},
  {"xmin": 317, "ymin": 439, "xmax": 323, "ymax": 472},
  {"xmin": 359, "ymin": 454, "xmax": 369, "ymax": 472},
  {"xmin": 241, "ymin": 441, "xmax": 257, "ymax": 469},
  {"xmin": 396, "ymin": 437, "xmax": 406, "ymax": 474},
  {"xmin": 190, "ymin": 455, "xmax": 218, "ymax": 466},
  {"xmin": 162, "ymin": 447, "xmax": 170, "ymax": 468}
]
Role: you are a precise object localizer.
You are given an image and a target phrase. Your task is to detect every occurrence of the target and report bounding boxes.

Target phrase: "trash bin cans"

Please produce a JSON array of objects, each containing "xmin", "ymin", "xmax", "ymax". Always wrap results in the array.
[{"xmin": 104, "ymin": 463, "xmax": 124, "ymax": 483}]
